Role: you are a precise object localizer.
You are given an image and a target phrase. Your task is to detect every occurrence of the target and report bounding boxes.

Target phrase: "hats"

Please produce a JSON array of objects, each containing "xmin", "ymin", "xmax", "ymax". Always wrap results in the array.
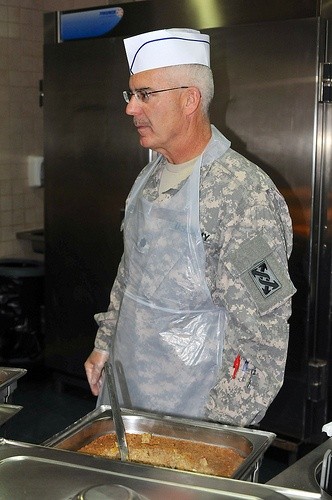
[{"xmin": 123, "ymin": 27, "xmax": 210, "ymax": 77}]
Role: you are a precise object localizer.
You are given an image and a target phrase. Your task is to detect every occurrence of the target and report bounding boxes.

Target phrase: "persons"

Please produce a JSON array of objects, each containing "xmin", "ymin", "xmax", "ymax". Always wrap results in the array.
[{"xmin": 85, "ymin": 29, "xmax": 294, "ymax": 427}]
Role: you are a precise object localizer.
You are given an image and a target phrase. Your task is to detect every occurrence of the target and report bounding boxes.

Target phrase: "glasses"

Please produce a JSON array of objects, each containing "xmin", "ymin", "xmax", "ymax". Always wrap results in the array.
[{"xmin": 123, "ymin": 87, "xmax": 191, "ymax": 104}]
